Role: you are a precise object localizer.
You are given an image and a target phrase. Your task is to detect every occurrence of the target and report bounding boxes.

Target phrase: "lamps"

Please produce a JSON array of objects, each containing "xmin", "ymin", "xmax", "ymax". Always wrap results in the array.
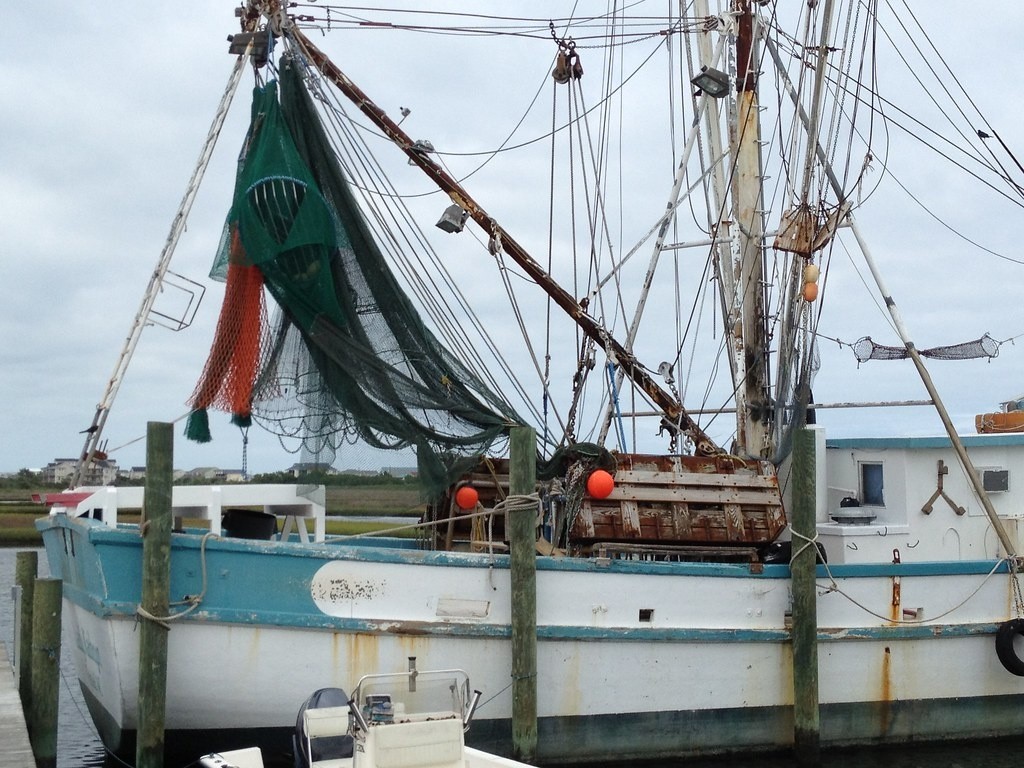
[
  {"xmin": 691, "ymin": 65, "xmax": 730, "ymax": 99},
  {"xmin": 227, "ymin": 30, "xmax": 274, "ymax": 60},
  {"xmin": 435, "ymin": 203, "xmax": 469, "ymax": 233}
]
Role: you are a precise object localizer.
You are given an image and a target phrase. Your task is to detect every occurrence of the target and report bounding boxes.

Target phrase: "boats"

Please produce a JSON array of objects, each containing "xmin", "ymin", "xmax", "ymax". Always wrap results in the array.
[
  {"xmin": 31, "ymin": 0, "xmax": 1023, "ymax": 761},
  {"xmin": 192, "ymin": 654, "xmax": 539, "ymax": 768}
]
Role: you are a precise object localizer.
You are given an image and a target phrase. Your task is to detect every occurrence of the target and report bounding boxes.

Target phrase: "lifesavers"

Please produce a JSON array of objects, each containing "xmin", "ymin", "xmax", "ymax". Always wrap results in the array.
[{"xmin": 994, "ymin": 616, "xmax": 1024, "ymax": 678}]
